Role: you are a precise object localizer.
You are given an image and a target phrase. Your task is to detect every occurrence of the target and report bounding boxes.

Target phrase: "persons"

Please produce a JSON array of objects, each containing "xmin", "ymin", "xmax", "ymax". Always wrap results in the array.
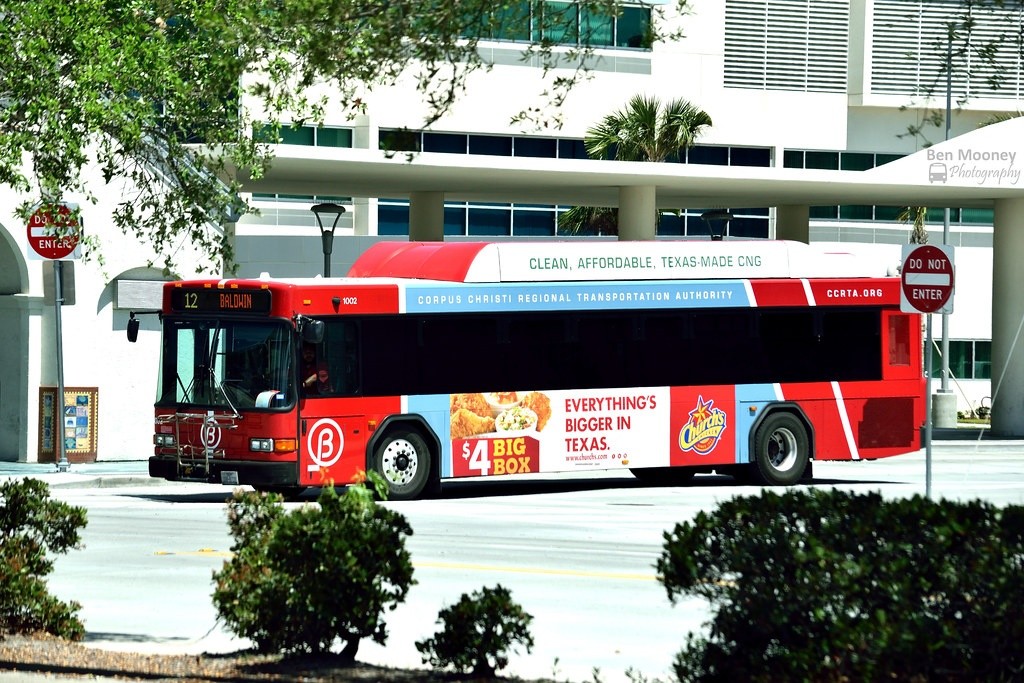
[{"xmin": 299, "ymin": 344, "xmax": 331, "ymax": 395}]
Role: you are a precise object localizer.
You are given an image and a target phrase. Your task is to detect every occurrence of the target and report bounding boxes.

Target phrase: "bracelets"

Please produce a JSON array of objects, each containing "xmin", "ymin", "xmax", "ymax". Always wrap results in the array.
[{"xmin": 303, "ymin": 381, "xmax": 307, "ymax": 388}]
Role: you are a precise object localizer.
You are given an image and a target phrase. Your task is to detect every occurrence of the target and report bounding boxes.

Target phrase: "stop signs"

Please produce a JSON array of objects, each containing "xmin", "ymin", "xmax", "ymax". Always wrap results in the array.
[
  {"xmin": 26, "ymin": 204, "xmax": 81, "ymax": 259},
  {"xmin": 902, "ymin": 244, "xmax": 955, "ymax": 314}
]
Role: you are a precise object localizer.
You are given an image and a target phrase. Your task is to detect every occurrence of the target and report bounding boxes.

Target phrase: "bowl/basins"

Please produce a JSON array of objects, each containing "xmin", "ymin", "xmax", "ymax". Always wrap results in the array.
[
  {"xmin": 494, "ymin": 408, "xmax": 537, "ymax": 434},
  {"xmin": 482, "ymin": 392, "xmax": 526, "ymax": 410}
]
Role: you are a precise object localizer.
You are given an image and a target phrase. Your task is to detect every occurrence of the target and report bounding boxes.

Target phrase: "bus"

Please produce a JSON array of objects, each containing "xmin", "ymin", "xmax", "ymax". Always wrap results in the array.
[{"xmin": 126, "ymin": 238, "xmax": 927, "ymax": 501}]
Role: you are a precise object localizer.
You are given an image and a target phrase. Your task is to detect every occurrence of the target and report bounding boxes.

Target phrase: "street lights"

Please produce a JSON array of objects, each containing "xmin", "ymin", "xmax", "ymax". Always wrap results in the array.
[{"xmin": 311, "ymin": 201, "xmax": 346, "ymax": 278}]
